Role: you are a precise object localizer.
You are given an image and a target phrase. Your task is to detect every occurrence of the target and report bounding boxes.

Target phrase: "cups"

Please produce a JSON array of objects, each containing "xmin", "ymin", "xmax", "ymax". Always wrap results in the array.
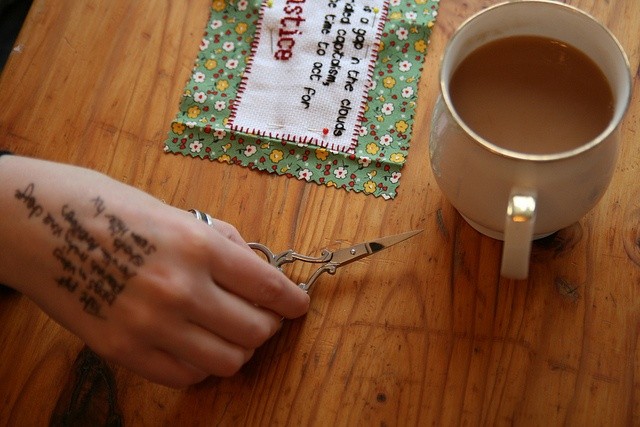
[{"xmin": 429, "ymin": 0, "xmax": 634, "ymax": 280}]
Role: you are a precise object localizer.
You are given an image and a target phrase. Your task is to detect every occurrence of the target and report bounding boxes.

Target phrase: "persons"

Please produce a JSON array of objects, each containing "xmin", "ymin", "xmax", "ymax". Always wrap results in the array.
[{"xmin": 1, "ymin": 146, "xmax": 313, "ymax": 390}]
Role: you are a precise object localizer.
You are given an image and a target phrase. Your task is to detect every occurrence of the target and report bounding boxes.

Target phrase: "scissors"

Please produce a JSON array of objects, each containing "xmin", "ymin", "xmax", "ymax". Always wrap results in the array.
[{"xmin": 246, "ymin": 230, "xmax": 424, "ymax": 322}]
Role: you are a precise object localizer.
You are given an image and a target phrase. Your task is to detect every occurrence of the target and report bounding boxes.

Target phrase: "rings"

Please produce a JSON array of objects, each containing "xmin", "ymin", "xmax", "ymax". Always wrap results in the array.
[{"xmin": 187, "ymin": 207, "xmax": 213, "ymax": 227}]
